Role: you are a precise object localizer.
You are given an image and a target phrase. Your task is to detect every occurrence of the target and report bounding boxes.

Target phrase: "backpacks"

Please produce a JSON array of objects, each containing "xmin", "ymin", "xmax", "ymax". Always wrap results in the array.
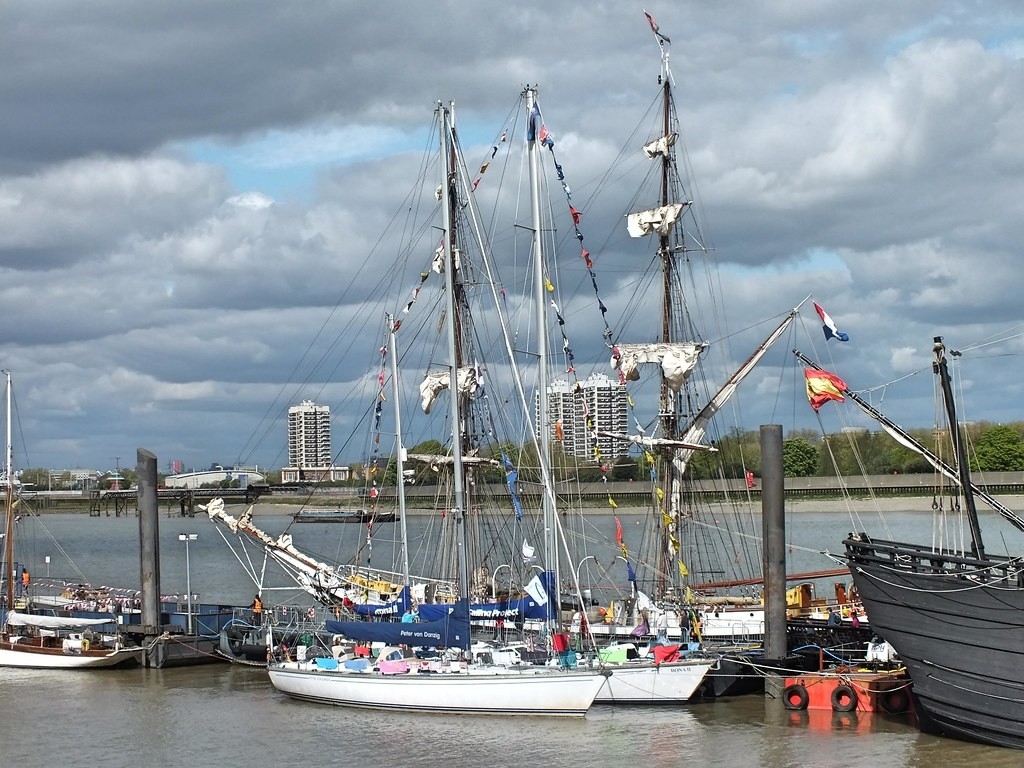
[{"xmin": 833, "ymin": 613, "xmax": 842, "ymax": 627}]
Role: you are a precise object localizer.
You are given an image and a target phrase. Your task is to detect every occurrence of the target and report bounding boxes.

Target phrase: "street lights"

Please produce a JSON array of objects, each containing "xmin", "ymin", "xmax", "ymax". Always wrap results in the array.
[{"xmin": 178, "ymin": 533, "xmax": 199, "ymax": 637}]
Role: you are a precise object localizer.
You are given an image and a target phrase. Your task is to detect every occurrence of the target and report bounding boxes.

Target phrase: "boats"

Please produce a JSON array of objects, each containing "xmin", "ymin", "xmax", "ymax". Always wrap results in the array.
[{"xmin": 290, "ymin": 505, "xmax": 395, "ymax": 523}]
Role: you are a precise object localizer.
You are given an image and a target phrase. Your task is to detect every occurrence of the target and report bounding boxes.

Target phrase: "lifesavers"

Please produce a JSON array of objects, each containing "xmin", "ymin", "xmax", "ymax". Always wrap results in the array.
[
  {"xmin": 830, "ymin": 686, "xmax": 857, "ymax": 712},
  {"xmin": 781, "ymin": 684, "xmax": 809, "ymax": 710}
]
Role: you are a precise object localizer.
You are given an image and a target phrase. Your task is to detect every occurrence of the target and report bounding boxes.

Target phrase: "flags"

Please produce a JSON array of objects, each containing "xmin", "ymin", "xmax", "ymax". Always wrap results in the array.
[
  {"xmin": 813, "ymin": 301, "xmax": 849, "ymax": 341},
  {"xmin": 804, "ymin": 368, "xmax": 849, "ymax": 413}
]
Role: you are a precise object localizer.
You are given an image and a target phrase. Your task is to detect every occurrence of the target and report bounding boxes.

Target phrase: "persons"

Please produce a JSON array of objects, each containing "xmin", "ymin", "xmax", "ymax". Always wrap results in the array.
[
  {"xmin": 21, "ymin": 568, "xmax": 30, "ymax": 597},
  {"xmin": 247, "ymin": 594, "xmax": 264, "ymax": 626},
  {"xmin": 492, "ymin": 597, "xmax": 505, "ymax": 640}
]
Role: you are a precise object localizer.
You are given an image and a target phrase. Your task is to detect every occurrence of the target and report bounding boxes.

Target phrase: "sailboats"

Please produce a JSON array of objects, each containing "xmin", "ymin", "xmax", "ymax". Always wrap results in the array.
[{"xmin": 0, "ymin": 15, "xmax": 1024, "ymax": 748}]
